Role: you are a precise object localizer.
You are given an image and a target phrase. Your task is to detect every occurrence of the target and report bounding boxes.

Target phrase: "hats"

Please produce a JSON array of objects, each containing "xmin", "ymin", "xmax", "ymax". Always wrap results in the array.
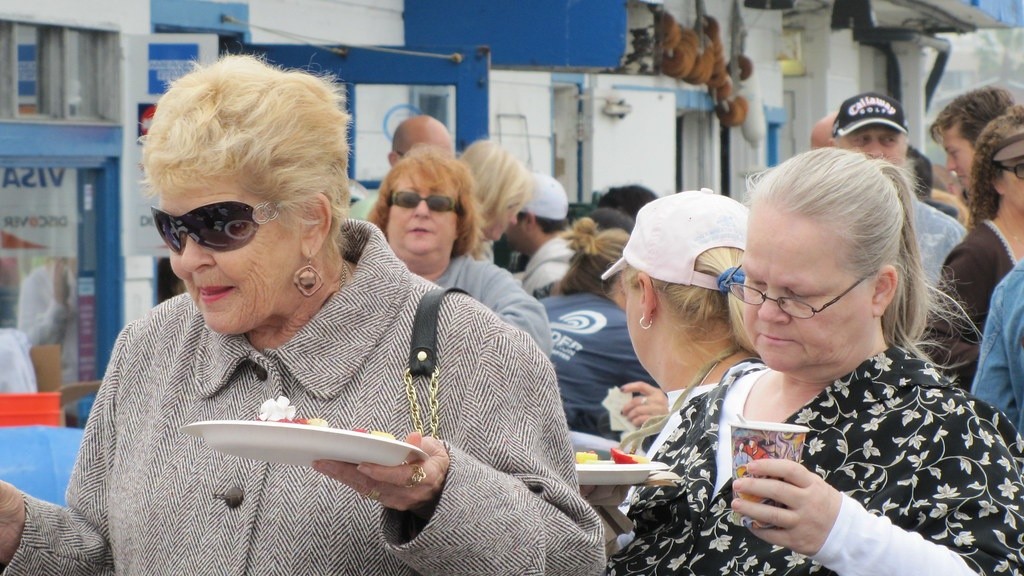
[
  {"xmin": 833, "ymin": 91, "xmax": 909, "ymax": 140},
  {"xmin": 602, "ymin": 188, "xmax": 749, "ymax": 291},
  {"xmin": 519, "ymin": 173, "xmax": 568, "ymax": 220},
  {"xmin": 994, "ymin": 130, "xmax": 1024, "ymax": 161}
]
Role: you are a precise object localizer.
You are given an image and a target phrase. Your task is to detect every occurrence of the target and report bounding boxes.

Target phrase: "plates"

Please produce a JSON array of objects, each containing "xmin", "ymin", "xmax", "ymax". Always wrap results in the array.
[
  {"xmin": 179, "ymin": 420, "xmax": 432, "ymax": 469},
  {"xmin": 574, "ymin": 459, "xmax": 671, "ymax": 485}
]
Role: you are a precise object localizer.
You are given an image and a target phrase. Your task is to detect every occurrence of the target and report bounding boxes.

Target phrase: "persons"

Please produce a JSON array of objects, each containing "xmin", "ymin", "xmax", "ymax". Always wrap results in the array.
[
  {"xmin": 608, "ymin": 149, "xmax": 1024, "ymax": 576},
  {"xmin": 0, "ymin": 49, "xmax": 607, "ymax": 576},
  {"xmin": 349, "ymin": 80, "xmax": 1023, "ymax": 514}
]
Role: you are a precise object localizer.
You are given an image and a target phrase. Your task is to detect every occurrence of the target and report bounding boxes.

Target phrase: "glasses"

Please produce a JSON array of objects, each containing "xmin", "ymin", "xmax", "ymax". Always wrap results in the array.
[
  {"xmin": 388, "ymin": 192, "xmax": 460, "ymax": 214},
  {"xmin": 724, "ymin": 265, "xmax": 876, "ymax": 319},
  {"xmin": 150, "ymin": 200, "xmax": 280, "ymax": 254},
  {"xmin": 1000, "ymin": 163, "xmax": 1024, "ymax": 180}
]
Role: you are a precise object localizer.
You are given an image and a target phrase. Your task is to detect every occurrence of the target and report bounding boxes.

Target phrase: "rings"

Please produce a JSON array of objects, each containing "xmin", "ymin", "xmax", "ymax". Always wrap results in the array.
[{"xmin": 642, "ymin": 396, "xmax": 648, "ymax": 405}]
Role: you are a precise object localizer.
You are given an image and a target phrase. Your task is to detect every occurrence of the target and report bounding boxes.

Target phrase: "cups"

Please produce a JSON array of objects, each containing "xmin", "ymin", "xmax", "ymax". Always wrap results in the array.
[{"xmin": 727, "ymin": 420, "xmax": 811, "ymax": 527}]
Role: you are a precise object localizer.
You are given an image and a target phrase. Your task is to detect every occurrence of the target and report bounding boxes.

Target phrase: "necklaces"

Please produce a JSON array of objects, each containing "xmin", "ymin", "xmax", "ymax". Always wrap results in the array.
[{"xmin": 699, "ymin": 361, "xmax": 720, "ymax": 387}]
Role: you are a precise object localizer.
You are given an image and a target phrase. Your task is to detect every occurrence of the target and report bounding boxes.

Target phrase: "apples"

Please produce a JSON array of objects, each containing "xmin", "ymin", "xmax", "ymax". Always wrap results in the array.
[{"xmin": 611, "ymin": 447, "xmax": 646, "ymax": 464}]
